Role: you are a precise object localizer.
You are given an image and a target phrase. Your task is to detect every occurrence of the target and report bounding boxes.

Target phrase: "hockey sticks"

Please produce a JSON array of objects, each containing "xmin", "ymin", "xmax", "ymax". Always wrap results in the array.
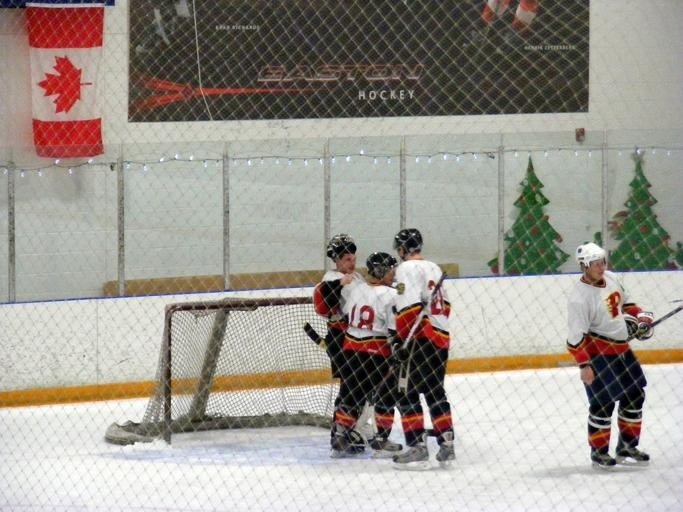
[
  {"xmin": 303, "ymin": 322, "xmax": 327, "ymax": 349},
  {"xmin": 369, "ymin": 272, "xmax": 447, "ymax": 405}
]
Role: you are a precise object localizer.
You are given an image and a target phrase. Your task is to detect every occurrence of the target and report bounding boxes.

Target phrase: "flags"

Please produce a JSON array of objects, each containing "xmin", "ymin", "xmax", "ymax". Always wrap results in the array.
[{"xmin": 25, "ymin": 1, "xmax": 104, "ymax": 159}]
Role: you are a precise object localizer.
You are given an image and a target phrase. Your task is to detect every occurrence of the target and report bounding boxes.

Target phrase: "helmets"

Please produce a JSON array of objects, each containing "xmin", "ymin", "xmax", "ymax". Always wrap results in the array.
[
  {"xmin": 574, "ymin": 239, "xmax": 609, "ymax": 273},
  {"xmin": 391, "ymin": 226, "xmax": 423, "ymax": 254},
  {"xmin": 324, "ymin": 230, "xmax": 359, "ymax": 261},
  {"xmin": 362, "ymin": 247, "xmax": 398, "ymax": 280}
]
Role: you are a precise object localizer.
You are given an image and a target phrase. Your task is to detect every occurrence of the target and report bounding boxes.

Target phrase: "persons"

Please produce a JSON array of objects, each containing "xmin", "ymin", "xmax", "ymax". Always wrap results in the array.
[
  {"xmin": 313, "ymin": 234, "xmax": 374, "ymax": 441},
  {"xmin": 459, "ymin": 0, "xmax": 540, "ymax": 56},
  {"xmin": 566, "ymin": 241, "xmax": 654, "ymax": 466},
  {"xmin": 392, "ymin": 228, "xmax": 456, "ymax": 464},
  {"xmin": 333, "ymin": 253, "xmax": 403, "ymax": 454}
]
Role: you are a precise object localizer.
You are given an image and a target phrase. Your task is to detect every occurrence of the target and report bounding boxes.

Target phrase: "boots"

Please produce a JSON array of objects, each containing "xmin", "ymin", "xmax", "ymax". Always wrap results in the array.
[
  {"xmin": 365, "ymin": 421, "xmax": 404, "ymax": 451},
  {"xmin": 390, "ymin": 432, "xmax": 430, "ymax": 467},
  {"xmin": 586, "ymin": 445, "xmax": 616, "ymax": 467},
  {"xmin": 431, "ymin": 430, "xmax": 455, "ymax": 463},
  {"xmin": 614, "ymin": 439, "xmax": 651, "ymax": 461},
  {"xmin": 327, "ymin": 413, "xmax": 365, "ymax": 453}
]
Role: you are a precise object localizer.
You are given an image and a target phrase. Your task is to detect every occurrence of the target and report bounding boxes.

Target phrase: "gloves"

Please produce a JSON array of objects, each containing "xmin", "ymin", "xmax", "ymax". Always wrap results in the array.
[
  {"xmin": 388, "ymin": 336, "xmax": 408, "ymax": 363},
  {"xmin": 634, "ymin": 309, "xmax": 655, "ymax": 340}
]
